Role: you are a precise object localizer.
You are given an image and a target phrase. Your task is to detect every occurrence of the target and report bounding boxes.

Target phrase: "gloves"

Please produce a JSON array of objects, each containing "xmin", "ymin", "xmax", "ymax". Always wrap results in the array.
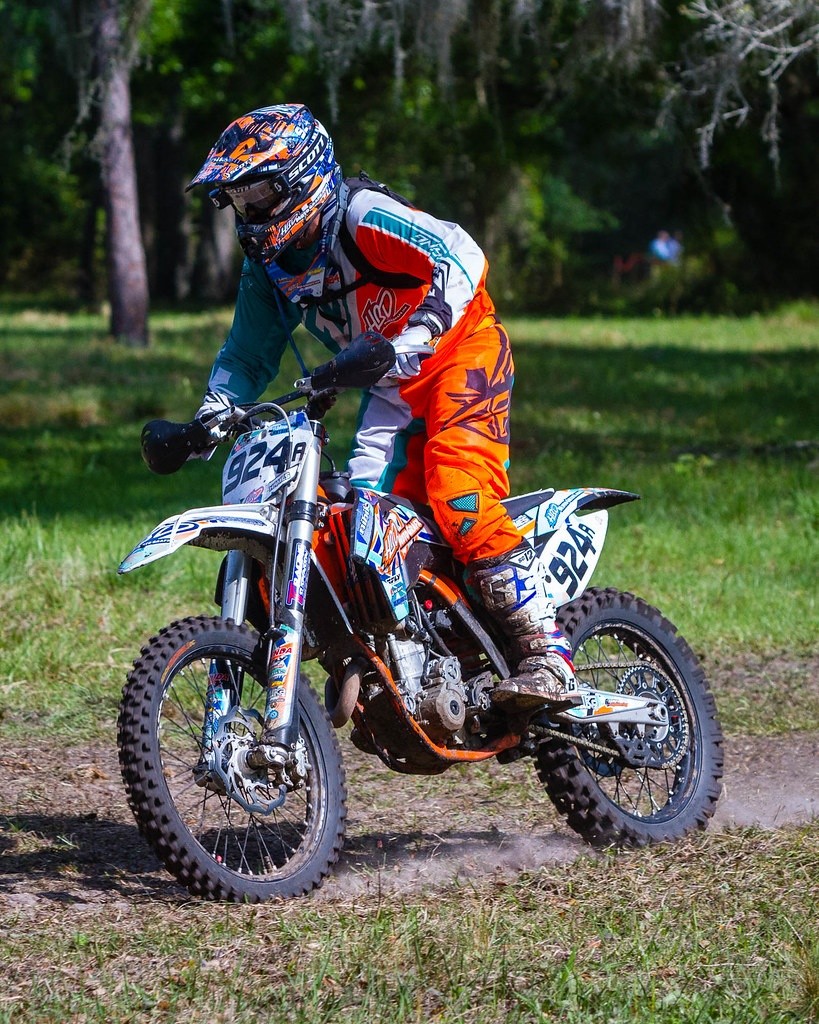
[
  {"xmin": 390, "ymin": 325, "xmax": 432, "ymax": 379},
  {"xmin": 195, "ymin": 391, "xmax": 234, "ymax": 445}
]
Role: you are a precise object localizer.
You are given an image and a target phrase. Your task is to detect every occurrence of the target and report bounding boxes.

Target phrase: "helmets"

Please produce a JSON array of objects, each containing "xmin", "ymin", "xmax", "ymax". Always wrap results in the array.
[{"xmin": 184, "ymin": 104, "xmax": 343, "ymax": 267}]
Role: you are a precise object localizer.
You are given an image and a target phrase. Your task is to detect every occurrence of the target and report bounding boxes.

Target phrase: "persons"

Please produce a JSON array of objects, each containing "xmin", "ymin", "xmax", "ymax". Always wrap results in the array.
[
  {"xmin": 652, "ymin": 220, "xmax": 682, "ymax": 266},
  {"xmin": 191, "ymin": 98, "xmax": 579, "ymax": 710}
]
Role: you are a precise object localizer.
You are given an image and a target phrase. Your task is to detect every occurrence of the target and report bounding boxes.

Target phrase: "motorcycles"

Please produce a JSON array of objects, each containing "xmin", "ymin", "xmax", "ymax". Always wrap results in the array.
[{"xmin": 115, "ymin": 332, "xmax": 725, "ymax": 903}]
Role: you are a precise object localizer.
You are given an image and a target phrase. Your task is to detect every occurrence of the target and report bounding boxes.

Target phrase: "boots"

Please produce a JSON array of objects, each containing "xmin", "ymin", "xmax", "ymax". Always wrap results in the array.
[
  {"xmin": 350, "ymin": 726, "xmax": 379, "ymax": 754},
  {"xmin": 468, "ymin": 536, "xmax": 578, "ymax": 710}
]
